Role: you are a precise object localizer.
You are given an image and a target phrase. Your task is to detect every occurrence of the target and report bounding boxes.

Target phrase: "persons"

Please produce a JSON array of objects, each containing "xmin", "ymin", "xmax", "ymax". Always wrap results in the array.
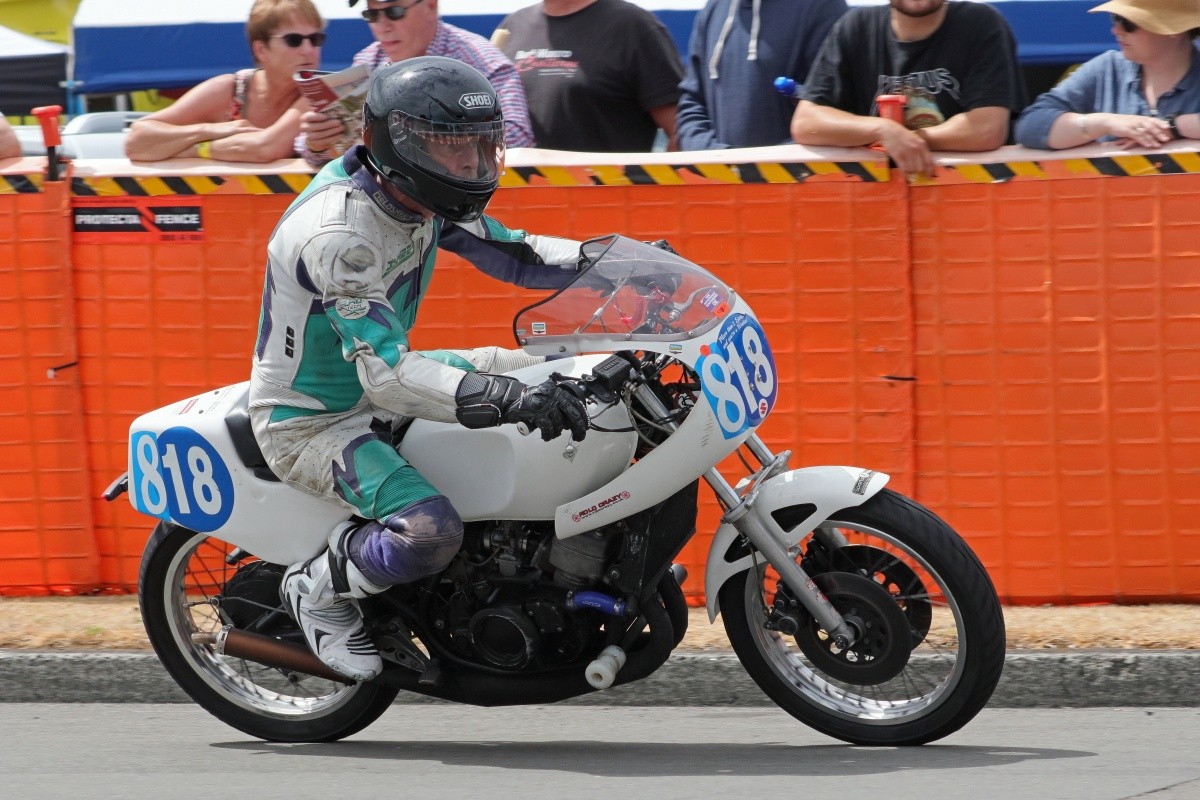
[
  {"xmin": 789, "ymin": 0, "xmax": 1014, "ymax": 178},
  {"xmin": 490, "ymin": 0, "xmax": 683, "ymax": 157},
  {"xmin": 677, "ymin": 0, "xmax": 851, "ymax": 150},
  {"xmin": 298, "ymin": 1, "xmax": 537, "ymax": 168},
  {"xmin": 0, "ymin": 110, "xmax": 23, "ymax": 160},
  {"xmin": 246, "ymin": 55, "xmax": 683, "ymax": 682},
  {"xmin": 1014, "ymin": 0, "xmax": 1200, "ymax": 150},
  {"xmin": 123, "ymin": 0, "xmax": 322, "ymax": 163}
]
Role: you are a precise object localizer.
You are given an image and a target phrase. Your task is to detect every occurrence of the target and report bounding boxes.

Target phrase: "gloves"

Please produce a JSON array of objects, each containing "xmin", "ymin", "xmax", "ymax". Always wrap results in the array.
[
  {"xmin": 576, "ymin": 240, "xmax": 683, "ymax": 298},
  {"xmin": 454, "ymin": 372, "xmax": 590, "ymax": 443}
]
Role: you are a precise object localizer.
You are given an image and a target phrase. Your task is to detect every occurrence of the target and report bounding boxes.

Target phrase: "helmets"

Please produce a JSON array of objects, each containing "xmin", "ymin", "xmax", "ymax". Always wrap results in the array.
[{"xmin": 364, "ymin": 55, "xmax": 506, "ymax": 223}]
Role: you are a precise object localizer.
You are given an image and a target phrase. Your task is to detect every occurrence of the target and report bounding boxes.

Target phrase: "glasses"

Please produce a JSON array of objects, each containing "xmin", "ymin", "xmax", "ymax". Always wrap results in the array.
[
  {"xmin": 1110, "ymin": 14, "xmax": 1136, "ymax": 33},
  {"xmin": 361, "ymin": 0, "xmax": 426, "ymax": 22},
  {"xmin": 255, "ymin": 32, "xmax": 327, "ymax": 48}
]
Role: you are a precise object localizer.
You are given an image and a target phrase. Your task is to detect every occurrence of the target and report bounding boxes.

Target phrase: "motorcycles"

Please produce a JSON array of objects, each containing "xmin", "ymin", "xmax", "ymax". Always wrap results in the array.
[{"xmin": 103, "ymin": 233, "xmax": 1007, "ymax": 744}]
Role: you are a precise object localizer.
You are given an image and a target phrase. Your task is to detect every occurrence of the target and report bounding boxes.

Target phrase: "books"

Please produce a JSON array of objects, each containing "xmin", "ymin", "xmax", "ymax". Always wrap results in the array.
[{"xmin": 292, "ymin": 64, "xmax": 374, "ymax": 145}]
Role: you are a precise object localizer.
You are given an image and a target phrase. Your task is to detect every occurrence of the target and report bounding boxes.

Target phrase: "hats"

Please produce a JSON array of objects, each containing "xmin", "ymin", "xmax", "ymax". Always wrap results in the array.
[{"xmin": 1086, "ymin": 0, "xmax": 1200, "ymax": 34}]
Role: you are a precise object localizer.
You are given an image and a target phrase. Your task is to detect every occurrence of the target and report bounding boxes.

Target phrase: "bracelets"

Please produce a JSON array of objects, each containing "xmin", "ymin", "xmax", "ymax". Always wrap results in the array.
[
  {"xmin": 308, "ymin": 147, "xmax": 329, "ymax": 153},
  {"xmin": 1166, "ymin": 110, "xmax": 1184, "ymax": 139},
  {"xmin": 197, "ymin": 140, "xmax": 212, "ymax": 159}
]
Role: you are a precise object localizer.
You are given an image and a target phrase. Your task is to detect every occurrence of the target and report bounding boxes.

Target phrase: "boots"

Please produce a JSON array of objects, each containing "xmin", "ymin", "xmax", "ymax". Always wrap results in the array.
[{"xmin": 279, "ymin": 521, "xmax": 392, "ymax": 681}]
